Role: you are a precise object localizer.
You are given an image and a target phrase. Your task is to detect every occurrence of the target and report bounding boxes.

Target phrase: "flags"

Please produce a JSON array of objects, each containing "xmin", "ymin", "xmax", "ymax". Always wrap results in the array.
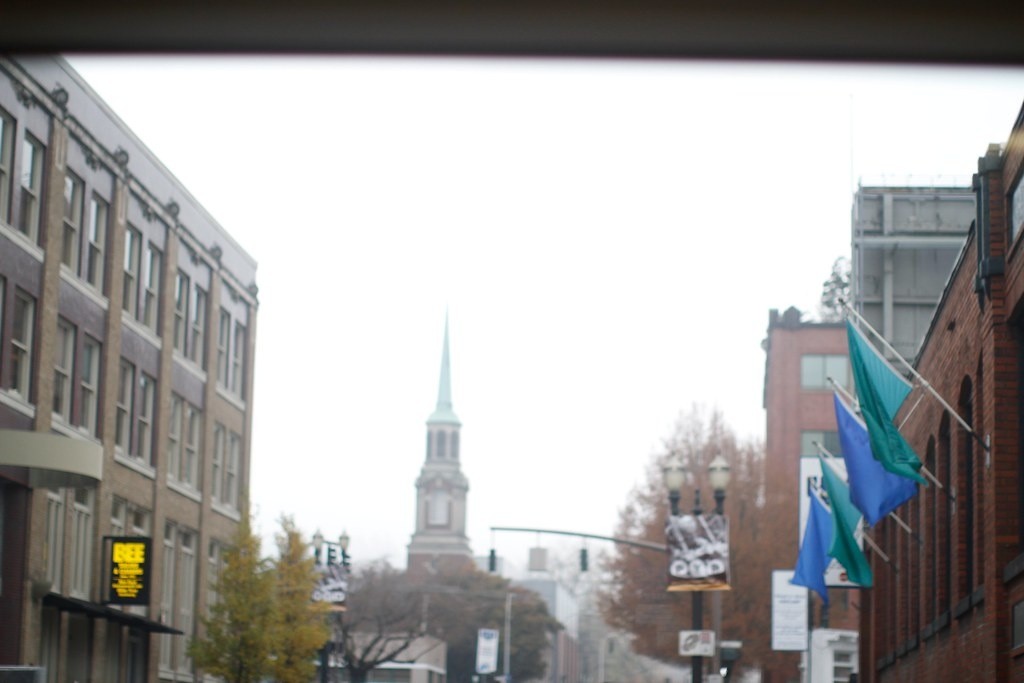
[
  {"xmin": 833, "ymin": 389, "xmax": 918, "ymax": 525},
  {"xmin": 818, "ymin": 451, "xmax": 874, "ymax": 588},
  {"xmin": 788, "ymin": 485, "xmax": 832, "ymax": 605},
  {"xmin": 845, "ymin": 314, "xmax": 928, "ymax": 486}
]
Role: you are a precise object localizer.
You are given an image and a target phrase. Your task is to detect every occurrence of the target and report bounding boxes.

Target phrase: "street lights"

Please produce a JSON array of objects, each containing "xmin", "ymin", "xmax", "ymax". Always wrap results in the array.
[
  {"xmin": 314, "ymin": 529, "xmax": 349, "ymax": 683},
  {"xmin": 666, "ymin": 453, "xmax": 732, "ymax": 683}
]
